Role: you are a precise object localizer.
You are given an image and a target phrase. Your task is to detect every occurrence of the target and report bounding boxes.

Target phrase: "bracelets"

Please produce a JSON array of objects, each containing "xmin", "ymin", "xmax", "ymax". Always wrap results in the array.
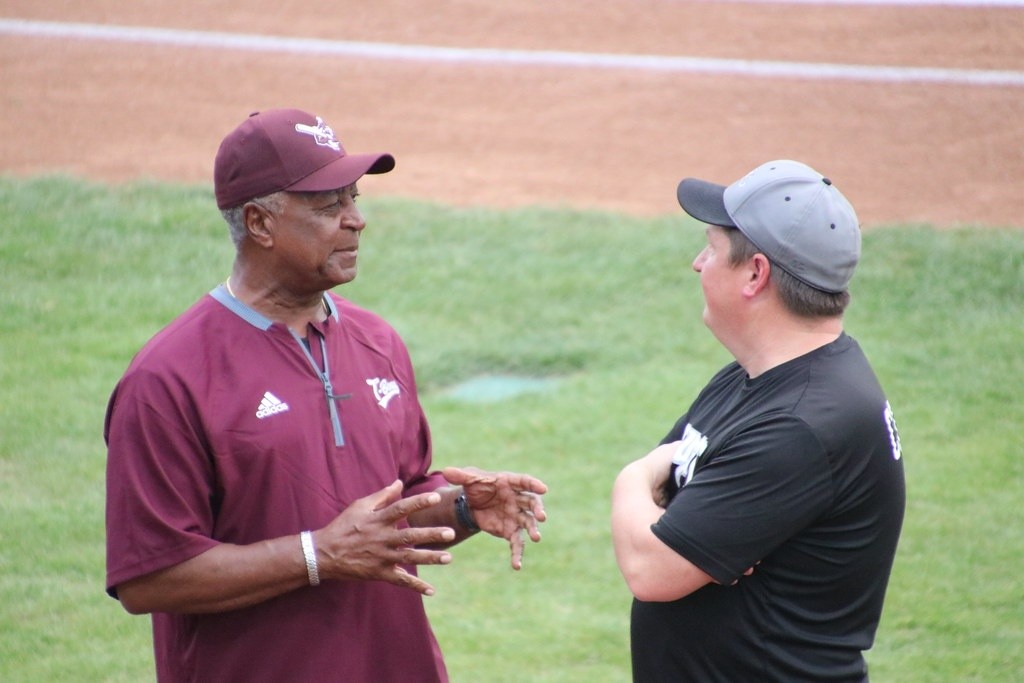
[
  {"xmin": 300, "ymin": 530, "xmax": 320, "ymax": 586},
  {"xmin": 454, "ymin": 493, "xmax": 480, "ymax": 534}
]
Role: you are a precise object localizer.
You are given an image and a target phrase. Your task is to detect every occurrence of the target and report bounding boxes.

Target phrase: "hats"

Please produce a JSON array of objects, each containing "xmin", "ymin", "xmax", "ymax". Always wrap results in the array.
[
  {"xmin": 214, "ymin": 108, "xmax": 395, "ymax": 210},
  {"xmin": 676, "ymin": 160, "xmax": 860, "ymax": 293}
]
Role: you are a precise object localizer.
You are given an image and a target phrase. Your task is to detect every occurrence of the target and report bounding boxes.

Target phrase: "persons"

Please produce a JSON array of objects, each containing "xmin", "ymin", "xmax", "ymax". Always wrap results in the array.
[
  {"xmin": 610, "ymin": 159, "xmax": 907, "ymax": 683},
  {"xmin": 103, "ymin": 107, "xmax": 549, "ymax": 683}
]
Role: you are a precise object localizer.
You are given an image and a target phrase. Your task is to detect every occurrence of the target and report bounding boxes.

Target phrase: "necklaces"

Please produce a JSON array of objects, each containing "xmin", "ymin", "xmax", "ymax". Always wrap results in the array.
[{"xmin": 226, "ymin": 275, "xmax": 330, "ymax": 318}]
